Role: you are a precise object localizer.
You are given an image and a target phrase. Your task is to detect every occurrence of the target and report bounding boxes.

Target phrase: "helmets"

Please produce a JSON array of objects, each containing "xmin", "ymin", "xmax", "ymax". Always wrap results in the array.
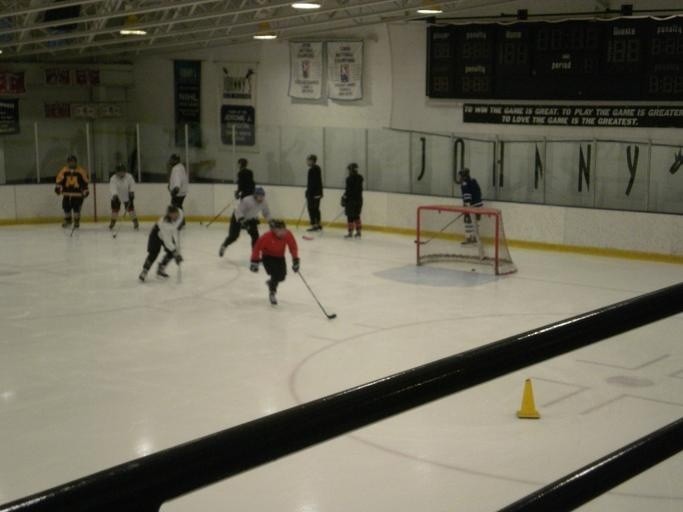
[
  {"xmin": 270, "ymin": 220, "xmax": 284, "ymax": 229},
  {"xmin": 255, "ymin": 188, "xmax": 264, "ymax": 196},
  {"xmin": 459, "ymin": 169, "xmax": 469, "ymax": 177},
  {"xmin": 168, "ymin": 206, "xmax": 177, "ymax": 212},
  {"xmin": 67, "ymin": 155, "xmax": 77, "ymax": 166},
  {"xmin": 114, "ymin": 164, "xmax": 125, "ymax": 173}
]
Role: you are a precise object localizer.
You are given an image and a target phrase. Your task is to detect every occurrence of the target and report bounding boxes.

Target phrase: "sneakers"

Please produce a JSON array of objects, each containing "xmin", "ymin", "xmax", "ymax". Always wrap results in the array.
[
  {"xmin": 269, "ymin": 293, "xmax": 276, "ymax": 304},
  {"xmin": 109, "ymin": 221, "xmax": 138, "ymax": 229},
  {"xmin": 345, "ymin": 233, "xmax": 361, "ymax": 237},
  {"xmin": 462, "ymin": 236, "xmax": 476, "ymax": 244},
  {"xmin": 140, "ymin": 270, "xmax": 167, "ymax": 280},
  {"xmin": 307, "ymin": 225, "xmax": 321, "ymax": 230},
  {"xmin": 62, "ymin": 221, "xmax": 79, "ymax": 228},
  {"xmin": 220, "ymin": 244, "xmax": 225, "ymax": 255}
]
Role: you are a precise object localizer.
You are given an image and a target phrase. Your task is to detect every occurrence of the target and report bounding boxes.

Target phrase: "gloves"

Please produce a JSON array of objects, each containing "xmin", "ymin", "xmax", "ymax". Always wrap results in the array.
[
  {"xmin": 250, "ymin": 263, "xmax": 258, "ymax": 272},
  {"xmin": 293, "ymin": 258, "xmax": 299, "ymax": 271},
  {"xmin": 175, "ymin": 254, "xmax": 182, "ymax": 262}
]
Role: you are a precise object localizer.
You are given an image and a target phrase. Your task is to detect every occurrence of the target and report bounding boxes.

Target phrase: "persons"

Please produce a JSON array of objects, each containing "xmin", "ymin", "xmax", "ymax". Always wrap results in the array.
[
  {"xmin": 247, "ymin": 217, "xmax": 301, "ymax": 306},
  {"xmin": 220, "ymin": 187, "xmax": 274, "ymax": 264},
  {"xmin": 135, "ymin": 206, "xmax": 185, "ymax": 282},
  {"xmin": 167, "ymin": 152, "xmax": 190, "ymax": 229},
  {"xmin": 304, "ymin": 154, "xmax": 327, "ymax": 232},
  {"xmin": 106, "ymin": 162, "xmax": 142, "ymax": 230},
  {"xmin": 455, "ymin": 168, "xmax": 487, "ymax": 243},
  {"xmin": 341, "ymin": 163, "xmax": 365, "ymax": 238},
  {"xmin": 53, "ymin": 154, "xmax": 91, "ymax": 229},
  {"xmin": 232, "ymin": 158, "xmax": 259, "ymax": 199}
]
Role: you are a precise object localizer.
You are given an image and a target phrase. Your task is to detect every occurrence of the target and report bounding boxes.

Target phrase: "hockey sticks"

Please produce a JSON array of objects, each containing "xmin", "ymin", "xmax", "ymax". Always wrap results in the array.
[
  {"xmin": 414, "ymin": 209, "xmax": 468, "ymax": 245},
  {"xmin": 111, "ymin": 198, "xmax": 134, "ymax": 239},
  {"xmin": 302, "ymin": 209, "xmax": 345, "ymax": 241},
  {"xmin": 292, "ymin": 266, "xmax": 337, "ymax": 321},
  {"xmin": 199, "ymin": 195, "xmax": 239, "ymax": 228}
]
